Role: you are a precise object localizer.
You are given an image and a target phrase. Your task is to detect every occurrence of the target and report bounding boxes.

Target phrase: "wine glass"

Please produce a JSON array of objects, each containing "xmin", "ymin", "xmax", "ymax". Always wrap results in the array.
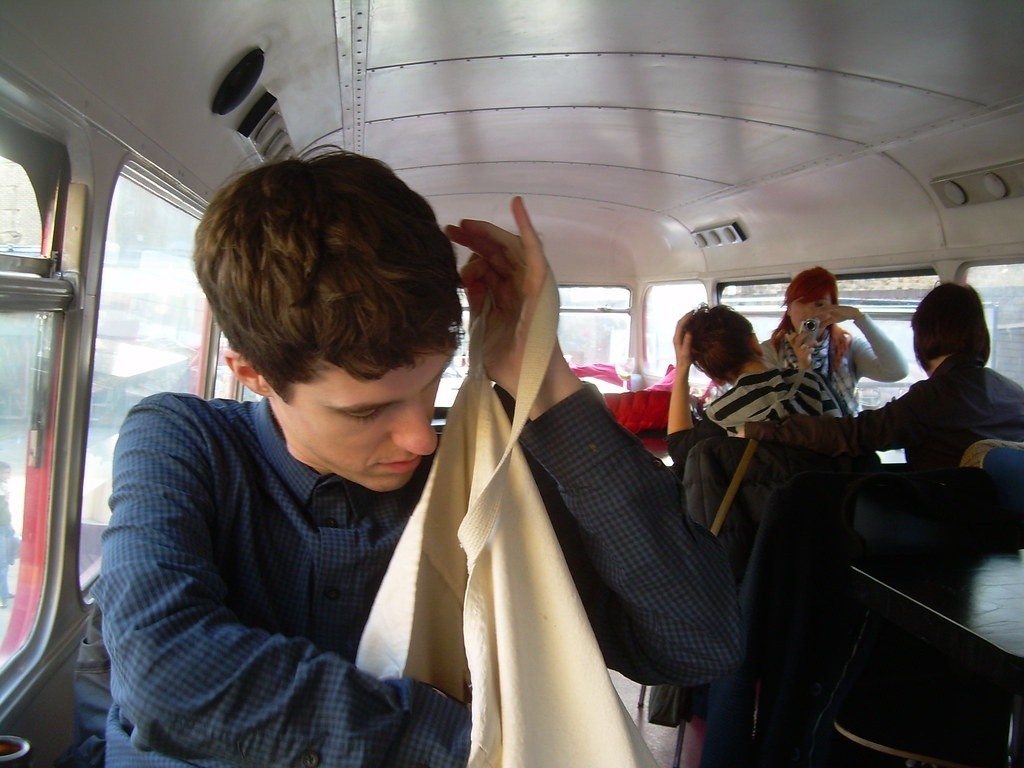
[{"xmin": 614, "ymin": 357, "xmax": 635, "ymax": 392}]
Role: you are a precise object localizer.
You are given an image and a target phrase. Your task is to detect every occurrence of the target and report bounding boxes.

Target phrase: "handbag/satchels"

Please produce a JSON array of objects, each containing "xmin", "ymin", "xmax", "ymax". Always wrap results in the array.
[
  {"xmin": 649, "ymin": 684, "xmax": 693, "ymax": 728},
  {"xmin": 354, "ymin": 260, "xmax": 664, "ymax": 768}
]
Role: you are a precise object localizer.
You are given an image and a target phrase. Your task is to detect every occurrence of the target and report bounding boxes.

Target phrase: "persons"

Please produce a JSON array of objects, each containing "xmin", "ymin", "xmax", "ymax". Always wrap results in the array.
[
  {"xmin": 663, "ymin": 265, "xmax": 906, "ymax": 464},
  {"xmin": 866, "ymin": 281, "xmax": 1024, "ymax": 465},
  {"xmin": 86, "ymin": 141, "xmax": 747, "ymax": 768}
]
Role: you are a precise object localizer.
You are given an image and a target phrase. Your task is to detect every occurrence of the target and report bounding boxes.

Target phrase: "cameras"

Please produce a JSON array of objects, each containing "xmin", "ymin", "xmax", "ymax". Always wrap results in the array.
[{"xmin": 799, "ymin": 317, "xmax": 820, "ymax": 340}]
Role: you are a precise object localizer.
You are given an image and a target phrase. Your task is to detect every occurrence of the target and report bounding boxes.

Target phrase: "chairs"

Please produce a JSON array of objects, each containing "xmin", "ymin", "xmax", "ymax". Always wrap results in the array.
[{"xmin": 598, "ymin": 390, "xmax": 1024, "ymax": 768}]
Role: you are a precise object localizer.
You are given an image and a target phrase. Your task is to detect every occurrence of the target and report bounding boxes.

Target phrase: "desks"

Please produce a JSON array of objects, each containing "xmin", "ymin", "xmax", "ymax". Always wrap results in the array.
[
  {"xmin": 639, "ymin": 438, "xmax": 674, "ymax": 466},
  {"xmin": 844, "ymin": 524, "xmax": 1024, "ymax": 694}
]
